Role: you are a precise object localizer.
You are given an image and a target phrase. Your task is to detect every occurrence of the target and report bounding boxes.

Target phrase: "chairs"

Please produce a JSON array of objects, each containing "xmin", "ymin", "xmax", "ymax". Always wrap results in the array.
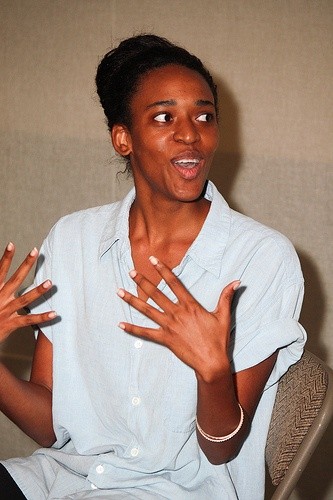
[{"xmin": 265, "ymin": 348, "xmax": 333, "ymax": 500}]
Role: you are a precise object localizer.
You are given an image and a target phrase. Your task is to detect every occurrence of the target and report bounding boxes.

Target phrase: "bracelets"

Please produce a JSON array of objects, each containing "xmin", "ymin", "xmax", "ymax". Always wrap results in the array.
[{"xmin": 195, "ymin": 403, "xmax": 244, "ymax": 442}]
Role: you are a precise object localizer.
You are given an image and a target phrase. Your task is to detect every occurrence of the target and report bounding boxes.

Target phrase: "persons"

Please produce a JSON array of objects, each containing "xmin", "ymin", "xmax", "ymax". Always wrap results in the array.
[{"xmin": 0, "ymin": 35, "xmax": 306, "ymax": 500}]
